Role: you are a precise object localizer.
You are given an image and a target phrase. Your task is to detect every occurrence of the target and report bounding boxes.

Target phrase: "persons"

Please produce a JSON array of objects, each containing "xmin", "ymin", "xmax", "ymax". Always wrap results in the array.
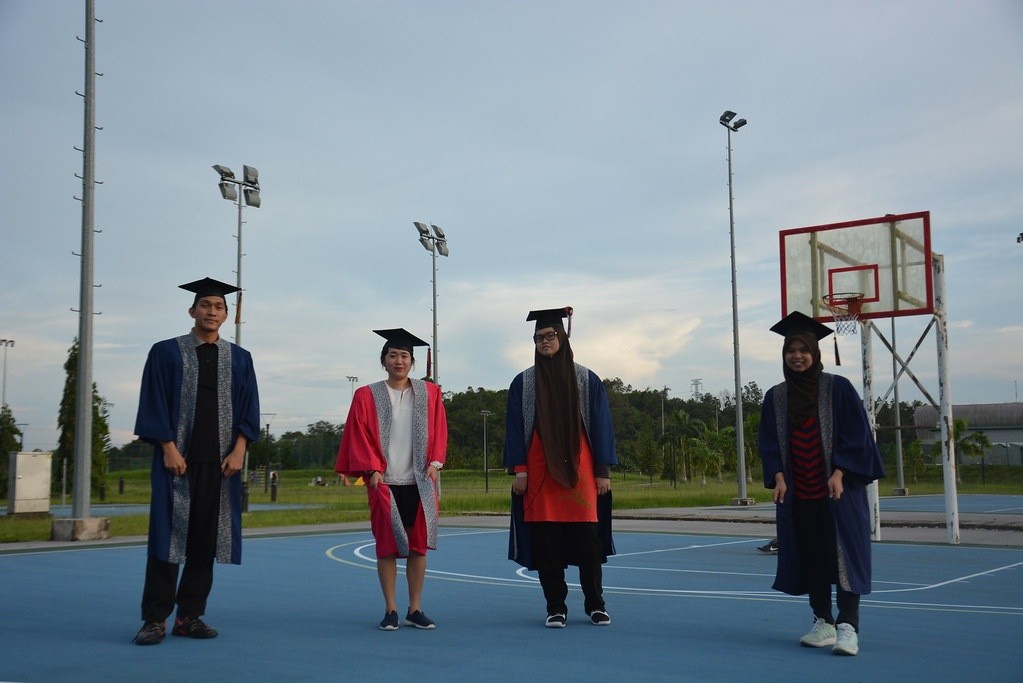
[
  {"xmin": 756, "ymin": 538, "xmax": 778, "ymax": 555},
  {"xmin": 271, "ymin": 472, "xmax": 277, "ymax": 502},
  {"xmin": 132, "ymin": 276, "xmax": 260, "ymax": 645},
  {"xmin": 758, "ymin": 309, "xmax": 886, "ymax": 656},
  {"xmin": 249, "ymin": 465, "xmax": 256, "ymax": 486},
  {"xmin": 500, "ymin": 306, "xmax": 618, "ymax": 628},
  {"xmin": 315, "ymin": 473, "xmax": 323, "ymax": 485},
  {"xmin": 335, "ymin": 328, "xmax": 448, "ymax": 630}
]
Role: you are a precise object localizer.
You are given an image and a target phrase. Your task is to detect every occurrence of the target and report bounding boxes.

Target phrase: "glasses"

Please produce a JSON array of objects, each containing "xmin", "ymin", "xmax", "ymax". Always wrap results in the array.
[{"xmin": 533, "ymin": 332, "xmax": 558, "ymax": 343}]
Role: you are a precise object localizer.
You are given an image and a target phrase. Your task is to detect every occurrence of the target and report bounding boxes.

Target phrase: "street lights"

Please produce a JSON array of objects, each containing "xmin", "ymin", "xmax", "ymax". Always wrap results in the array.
[
  {"xmin": 213, "ymin": 163, "xmax": 263, "ymax": 344},
  {"xmin": 345, "ymin": 375, "xmax": 359, "ymax": 397},
  {"xmin": 260, "ymin": 413, "xmax": 276, "ymax": 495},
  {"xmin": 412, "ymin": 221, "xmax": 450, "ymax": 385},
  {"xmin": 480, "ymin": 410, "xmax": 491, "ymax": 494},
  {"xmin": 717, "ymin": 108, "xmax": 754, "ymax": 504},
  {"xmin": 0, "ymin": 338, "xmax": 15, "ymax": 413}
]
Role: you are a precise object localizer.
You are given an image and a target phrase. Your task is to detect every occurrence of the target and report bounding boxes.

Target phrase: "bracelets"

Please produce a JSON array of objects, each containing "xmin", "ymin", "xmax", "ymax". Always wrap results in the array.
[{"xmin": 370, "ymin": 470, "xmax": 377, "ymax": 477}]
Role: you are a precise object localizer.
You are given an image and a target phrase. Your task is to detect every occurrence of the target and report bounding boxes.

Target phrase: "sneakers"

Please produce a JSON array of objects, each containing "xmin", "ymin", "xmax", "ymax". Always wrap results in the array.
[
  {"xmin": 545, "ymin": 613, "xmax": 566, "ymax": 627},
  {"xmin": 380, "ymin": 610, "xmax": 399, "ymax": 630},
  {"xmin": 833, "ymin": 623, "xmax": 858, "ymax": 655},
  {"xmin": 756, "ymin": 540, "xmax": 778, "ymax": 552},
  {"xmin": 590, "ymin": 610, "xmax": 611, "ymax": 625},
  {"xmin": 800, "ymin": 613, "xmax": 837, "ymax": 647},
  {"xmin": 404, "ymin": 606, "xmax": 436, "ymax": 628},
  {"xmin": 131, "ymin": 622, "xmax": 165, "ymax": 644},
  {"xmin": 172, "ymin": 616, "xmax": 217, "ymax": 638}
]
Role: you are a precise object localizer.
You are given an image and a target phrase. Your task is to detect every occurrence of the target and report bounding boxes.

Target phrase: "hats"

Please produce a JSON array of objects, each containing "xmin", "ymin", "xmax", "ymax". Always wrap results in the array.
[
  {"xmin": 769, "ymin": 311, "xmax": 840, "ymax": 366},
  {"xmin": 372, "ymin": 328, "xmax": 431, "ymax": 377},
  {"xmin": 178, "ymin": 277, "xmax": 243, "ymax": 324},
  {"xmin": 526, "ymin": 306, "xmax": 573, "ymax": 337}
]
